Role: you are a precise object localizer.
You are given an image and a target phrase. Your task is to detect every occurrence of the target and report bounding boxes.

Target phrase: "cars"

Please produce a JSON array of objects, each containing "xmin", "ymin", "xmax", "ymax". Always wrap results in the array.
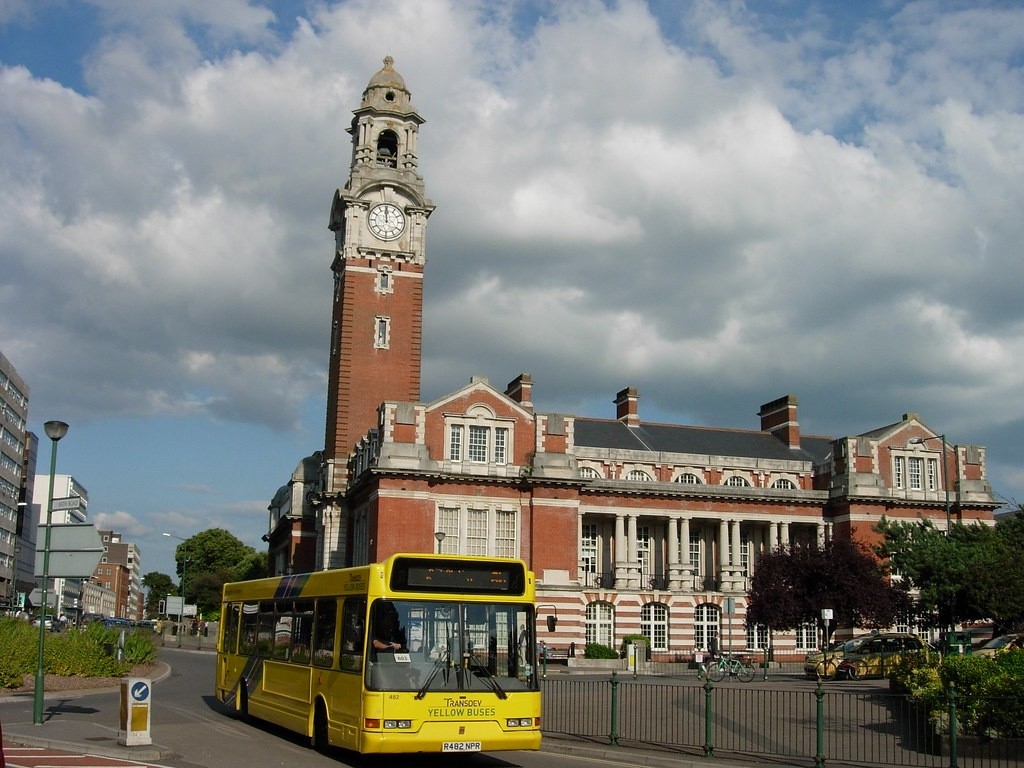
[
  {"xmin": 32, "ymin": 614, "xmax": 62, "ymax": 633},
  {"xmin": 80, "ymin": 614, "xmax": 156, "ymax": 628},
  {"xmin": 971, "ymin": 633, "xmax": 1024, "ymax": 661},
  {"xmin": 802, "ymin": 633, "xmax": 941, "ymax": 680}
]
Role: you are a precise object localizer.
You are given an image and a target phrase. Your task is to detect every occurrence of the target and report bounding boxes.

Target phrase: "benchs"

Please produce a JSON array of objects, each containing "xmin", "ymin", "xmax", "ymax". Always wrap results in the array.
[{"xmin": 538, "ymin": 648, "xmax": 571, "ymax": 660}]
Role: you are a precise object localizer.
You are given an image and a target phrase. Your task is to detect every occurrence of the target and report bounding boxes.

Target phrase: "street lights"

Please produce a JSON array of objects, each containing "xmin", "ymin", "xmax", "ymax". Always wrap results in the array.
[
  {"xmin": 433, "ymin": 532, "xmax": 446, "ymax": 553},
  {"xmin": 161, "ymin": 532, "xmax": 188, "ymax": 650},
  {"xmin": 908, "ymin": 433, "xmax": 952, "ymax": 535},
  {"xmin": 31, "ymin": 420, "xmax": 70, "ymax": 725}
]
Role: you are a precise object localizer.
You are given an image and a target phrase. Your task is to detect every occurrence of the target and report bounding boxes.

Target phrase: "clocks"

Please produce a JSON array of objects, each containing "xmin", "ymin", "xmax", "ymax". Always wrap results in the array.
[{"xmin": 366, "ymin": 202, "xmax": 407, "ymax": 242}]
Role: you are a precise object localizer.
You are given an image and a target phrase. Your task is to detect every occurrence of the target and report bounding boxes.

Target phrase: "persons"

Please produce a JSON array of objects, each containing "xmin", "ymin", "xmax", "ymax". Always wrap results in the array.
[
  {"xmin": 537, "ymin": 640, "xmax": 545, "ymax": 656},
  {"xmin": 190, "ymin": 620, "xmax": 209, "ymax": 637},
  {"xmin": 60, "ymin": 615, "xmax": 67, "ymax": 628},
  {"xmin": 710, "ymin": 631, "xmax": 723, "ymax": 651},
  {"xmin": 374, "ymin": 619, "xmax": 401, "ymax": 652}
]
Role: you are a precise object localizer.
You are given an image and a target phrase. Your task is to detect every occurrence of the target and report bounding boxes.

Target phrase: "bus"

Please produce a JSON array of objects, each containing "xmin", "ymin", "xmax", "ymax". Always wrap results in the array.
[{"xmin": 216, "ymin": 551, "xmax": 558, "ymax": 759}]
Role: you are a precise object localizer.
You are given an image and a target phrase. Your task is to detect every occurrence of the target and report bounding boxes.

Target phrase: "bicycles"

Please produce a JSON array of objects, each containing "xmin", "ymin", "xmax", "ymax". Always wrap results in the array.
[
  {"xmin": 816, "ymin": 650, "xmax": 869, "ymax": 681},
  {"xmin": 707, "ymin": 652, "xmax": 756, "ymax": 683}
]
